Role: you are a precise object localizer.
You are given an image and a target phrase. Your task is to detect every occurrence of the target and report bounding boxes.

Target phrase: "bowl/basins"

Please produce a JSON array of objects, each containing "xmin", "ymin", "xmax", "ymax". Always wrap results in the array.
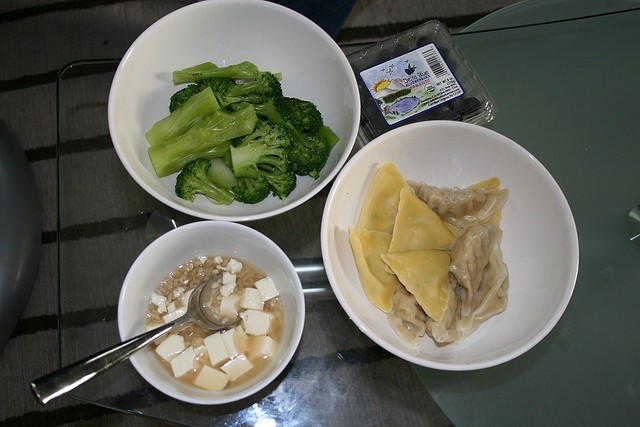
[
  {"xmin": 105, "ymin": 1, "xmax": 363, "ymax": 222},
  {"xmin": 319, "ymin": 118, "xmax": 582, "ymax": 374},
  {"xmin": 114, "ymin": 219, "xmax": 307, "ymax": 406}
]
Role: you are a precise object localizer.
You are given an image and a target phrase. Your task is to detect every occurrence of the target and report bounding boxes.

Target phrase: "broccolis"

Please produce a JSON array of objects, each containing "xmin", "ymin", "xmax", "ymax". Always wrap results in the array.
[{"xmin": 147, "ymin": 61, "xmax": 340, "ymax": 202}]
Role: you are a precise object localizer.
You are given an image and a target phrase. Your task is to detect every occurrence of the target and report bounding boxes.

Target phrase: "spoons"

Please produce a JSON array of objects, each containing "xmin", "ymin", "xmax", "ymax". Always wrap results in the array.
[{"xmin": 28, "ymin": 271, "xmax": 243, "ymax": 406}]
